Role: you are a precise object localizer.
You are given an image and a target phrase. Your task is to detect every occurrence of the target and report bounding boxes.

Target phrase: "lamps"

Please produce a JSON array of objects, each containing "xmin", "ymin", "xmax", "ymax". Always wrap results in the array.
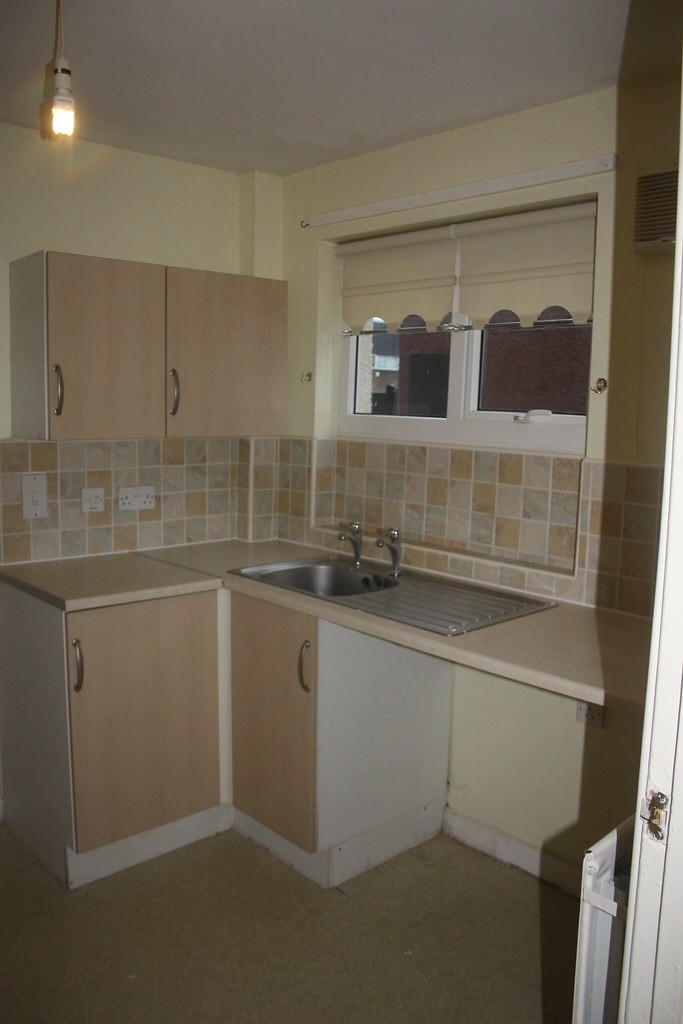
[{"xmin": 52, "ymin": 0, "xmax": 74, "ymax": 136}]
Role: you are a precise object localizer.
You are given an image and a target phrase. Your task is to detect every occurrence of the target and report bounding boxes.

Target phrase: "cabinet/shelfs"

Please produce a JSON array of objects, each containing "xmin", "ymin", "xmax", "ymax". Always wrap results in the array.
[
  {"xmin": 0, "ymin": 551, "xmax": 231, "ymax": 890},
  {"xmin": 10, "ymin": 249, "xmax": 288, "ymax": 436},
  {"xmin": 223, "ymin": 572, "xmax": 455, "ymax": 891}
]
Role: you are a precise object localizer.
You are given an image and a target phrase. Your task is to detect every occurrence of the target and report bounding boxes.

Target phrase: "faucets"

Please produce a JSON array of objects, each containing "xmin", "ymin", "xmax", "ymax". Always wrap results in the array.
[
  {"xmin": 339, "ymin": 521, "xmax": 362, "ymax": 564},
  {"xmin": 377, "ymin": 528, "xmax": 401, "ymax": 574}
]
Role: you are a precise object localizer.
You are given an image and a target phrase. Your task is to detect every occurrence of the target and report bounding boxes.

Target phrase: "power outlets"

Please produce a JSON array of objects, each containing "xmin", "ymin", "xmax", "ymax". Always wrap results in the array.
[
  {"xmin": 576, "ymin": 699, "xmax": 607, "ymax": 728},
  {"xmin": 118, "ymin": 486, "xmax": 155, "ymax": 510},
  {"xmin": 82, "ymin": 488, "xmax": 103, "ymax": 512}
]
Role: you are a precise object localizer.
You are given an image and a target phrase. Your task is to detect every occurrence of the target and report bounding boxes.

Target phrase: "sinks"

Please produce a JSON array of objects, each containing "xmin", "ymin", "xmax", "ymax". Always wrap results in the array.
[{"xmin": 248, "ymin": 563, "xmax": 401, "ymax": 602}]
